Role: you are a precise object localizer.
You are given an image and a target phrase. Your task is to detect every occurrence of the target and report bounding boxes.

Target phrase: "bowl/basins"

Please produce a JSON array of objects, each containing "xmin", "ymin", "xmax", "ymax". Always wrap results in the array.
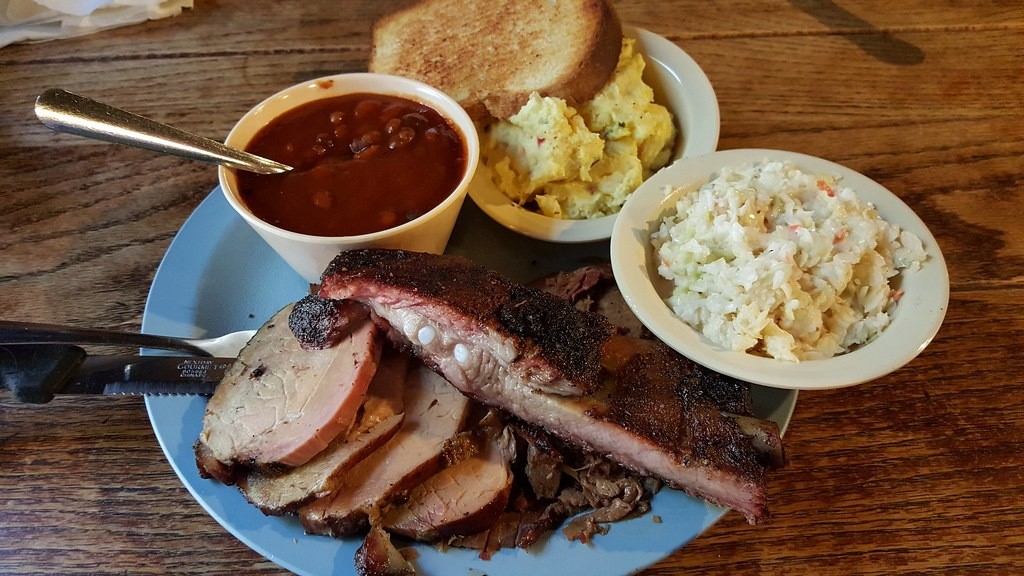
[{"xmin": 218, "ymin": 72, "xmax": 479, "ymax": 286}]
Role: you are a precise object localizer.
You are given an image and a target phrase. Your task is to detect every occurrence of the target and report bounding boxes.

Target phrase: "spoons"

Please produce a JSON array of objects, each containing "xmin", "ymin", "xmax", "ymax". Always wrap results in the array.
[{"xmin": 0, "ymin": 320, "xmax": 258, "ymax": 358}]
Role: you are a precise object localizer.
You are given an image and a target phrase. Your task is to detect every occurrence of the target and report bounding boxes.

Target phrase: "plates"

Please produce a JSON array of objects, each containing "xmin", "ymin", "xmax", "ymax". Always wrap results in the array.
[
  {"xmin": 611, "ymin": 149, "xmax": 951, "ymax": 390},
  {"xmin": 468, "ymin": 26, "xmax": 721, "ymax": 241},
  {"xmin": 140, "ymin": 185, "xmax": 799, "ymax": 576}
]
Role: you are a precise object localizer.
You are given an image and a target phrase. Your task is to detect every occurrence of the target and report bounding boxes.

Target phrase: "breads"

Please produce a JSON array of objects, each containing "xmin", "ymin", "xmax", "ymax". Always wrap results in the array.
[{"xmin": 365, "ymin": 0, "xmax": 623, "ymax": 123}]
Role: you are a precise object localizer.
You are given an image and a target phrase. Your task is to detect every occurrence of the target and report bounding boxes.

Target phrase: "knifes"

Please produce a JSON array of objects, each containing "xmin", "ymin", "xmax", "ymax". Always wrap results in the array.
[{"xmin": 0, "ymin": 344, "xmax": 238, "ymax": 405}]
